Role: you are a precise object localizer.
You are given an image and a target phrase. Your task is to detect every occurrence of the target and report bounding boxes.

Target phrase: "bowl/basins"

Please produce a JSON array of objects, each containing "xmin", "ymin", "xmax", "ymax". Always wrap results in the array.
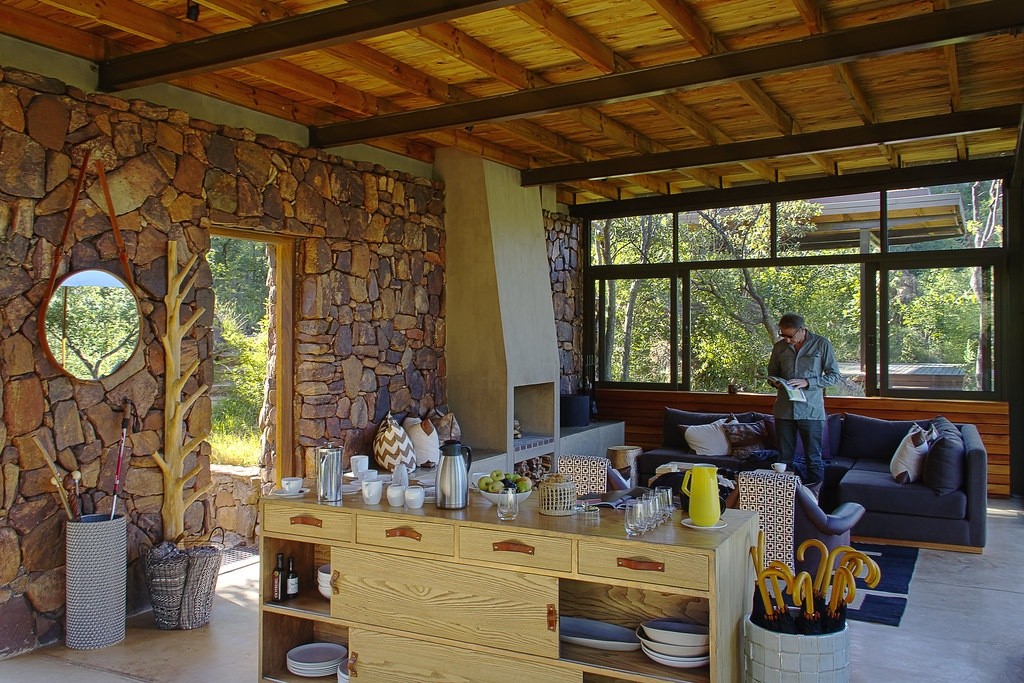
[
  {"xmin": 318, "ymin": 563, "xmax": 330, "ymax": 599},
  {"xmin": 481, "ymin": 489, "xmax": 532, "ymax": 506}
]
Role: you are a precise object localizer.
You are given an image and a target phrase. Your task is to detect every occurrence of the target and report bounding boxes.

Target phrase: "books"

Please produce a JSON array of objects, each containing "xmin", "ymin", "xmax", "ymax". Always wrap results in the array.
[{"xmin": 767, "ymin": 375, "xmax": 806, "ymax": 403}]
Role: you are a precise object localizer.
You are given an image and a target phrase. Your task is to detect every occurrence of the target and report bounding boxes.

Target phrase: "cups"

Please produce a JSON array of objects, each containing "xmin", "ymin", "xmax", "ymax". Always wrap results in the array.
[
  {"xmin": 560, "ymin": 394, "xmax": 590, "ymax": 427},
  {"xmin": 497, "ymin": 488, "xmax": 518, "ymax": 520},
  {"xmin": 771, "ymin": 462, "xmax": 786, "ymax": 472},
  {"xmin": 350, "ymin": 455, "xmax": 426, "ymax": 509},
  {"xmin": 282, "ymin": 476, "xmax": 303, "ymax": 494},
  {"xmin": 729, "ymin": 384, "xmax": 737, "ymax": 394},
  {"xmin": 624, "ymin": 486, "xmax": 673, "ymax": 537},
  {"xmin": 316, "ymin": 445, "xmax": 342, "ymax": 502}
]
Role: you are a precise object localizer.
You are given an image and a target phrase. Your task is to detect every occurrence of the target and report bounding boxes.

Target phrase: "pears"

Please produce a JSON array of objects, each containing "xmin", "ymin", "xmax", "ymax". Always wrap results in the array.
[{"xmin": 504, "ymin": 472, "xmax": 532, "ymax": 492}]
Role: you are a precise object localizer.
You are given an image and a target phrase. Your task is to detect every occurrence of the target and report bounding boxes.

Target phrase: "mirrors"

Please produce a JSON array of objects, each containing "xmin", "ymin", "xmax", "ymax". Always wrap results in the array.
[{"xmin": 40, "ymin": 267, "xmax": 142, "ymax": 386}]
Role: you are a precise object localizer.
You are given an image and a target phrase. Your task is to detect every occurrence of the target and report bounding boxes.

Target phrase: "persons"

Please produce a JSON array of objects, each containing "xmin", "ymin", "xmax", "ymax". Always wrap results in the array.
[{"xmin": 767, "ymin": 312, "xmax": 841, "ymax": 510}]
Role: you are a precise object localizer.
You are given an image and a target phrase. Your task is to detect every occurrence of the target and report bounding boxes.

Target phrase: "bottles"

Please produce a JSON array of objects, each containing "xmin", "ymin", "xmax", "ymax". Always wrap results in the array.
[{"xmin": 272, "ymin": 553, "xmax": 299, "ymax": 603}]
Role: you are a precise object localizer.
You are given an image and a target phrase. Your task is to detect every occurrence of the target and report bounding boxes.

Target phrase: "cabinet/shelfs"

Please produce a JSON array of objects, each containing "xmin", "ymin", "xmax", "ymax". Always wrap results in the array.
[{"xmin": 255, "ymin": 468, "xmax": 761, "ymax": 683}]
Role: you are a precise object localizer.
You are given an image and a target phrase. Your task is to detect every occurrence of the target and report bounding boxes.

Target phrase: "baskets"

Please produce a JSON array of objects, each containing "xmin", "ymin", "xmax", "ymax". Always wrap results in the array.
[
  {"xmin": 538, "ymin": 480, "xmax": 578, "ymax": 516},
  {"xmin": 140, "ymin": 527, "xmax": 224, "ymax": 631}
]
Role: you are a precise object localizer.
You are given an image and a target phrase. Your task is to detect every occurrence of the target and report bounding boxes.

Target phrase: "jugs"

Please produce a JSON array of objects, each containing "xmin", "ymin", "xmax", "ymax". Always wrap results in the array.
[
  {"xmin": 434, "ymin": 439, "xmax": 472, "ymax": 508},
  {"xmin": 682, "ymin": 464, "xmax": 720, "ymax": 527}
]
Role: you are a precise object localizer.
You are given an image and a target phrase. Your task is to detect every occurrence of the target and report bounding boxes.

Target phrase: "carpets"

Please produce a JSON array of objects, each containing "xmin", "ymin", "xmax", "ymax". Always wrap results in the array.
[{"xmin": 763, "ymin": 541, "xmax": 919, "ymax": 628}]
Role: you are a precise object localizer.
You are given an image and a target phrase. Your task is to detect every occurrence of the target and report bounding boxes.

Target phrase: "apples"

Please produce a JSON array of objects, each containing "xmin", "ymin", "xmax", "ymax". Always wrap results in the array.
[{"xmin": 478, "ymin": 470, "xmax": 506, "ymax": 494}]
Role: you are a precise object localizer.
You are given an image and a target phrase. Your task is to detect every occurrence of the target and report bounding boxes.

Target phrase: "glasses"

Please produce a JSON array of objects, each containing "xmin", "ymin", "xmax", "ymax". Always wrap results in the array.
[{"xmin": 778, "ymin": 327, "xmax": 801, "ymax": 339}]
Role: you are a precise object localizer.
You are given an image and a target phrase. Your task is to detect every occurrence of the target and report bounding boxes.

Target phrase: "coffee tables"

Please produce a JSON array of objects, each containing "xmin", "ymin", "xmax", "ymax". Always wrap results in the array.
[{"xmin": 647, "ymin": 464, "xmax": 740, "ymax": 509}]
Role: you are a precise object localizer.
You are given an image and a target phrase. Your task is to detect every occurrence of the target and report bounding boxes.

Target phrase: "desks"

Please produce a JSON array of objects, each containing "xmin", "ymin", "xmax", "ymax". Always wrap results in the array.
[
  {"xmin": 558, "ymin": 421, "xmax": 628, "ymax": 459},
  {"xmin": 343, "ymin": 445, "xmax": 508, "ymax": 489}
]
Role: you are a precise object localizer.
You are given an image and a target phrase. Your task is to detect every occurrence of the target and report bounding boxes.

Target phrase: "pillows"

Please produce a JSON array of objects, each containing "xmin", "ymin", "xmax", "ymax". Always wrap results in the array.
[
  {"xmin": 403, "ymin": 411, "xmax": 440, "ymax": 468},
  {"xmin": 841, "ymin": 411, "xmax": 934, "ymax": 460},
  {"xmin": 721, "ymin": 418, "xmax": 770, "ymax": 461},
  {"xmin": 889, "ymin": 429, "xmax": 930, "ymax": 484},
  {"xmin": 372, "ymin": 410, "xmax": 416, "ymax": 475},
  {"xmin": 424, "ymin": 407, "xmax": 461, "ymax": 461},
  {"xmin": 773, "ymin": 413, "xmax": 833, "ymax": 460},
  {"xmin": 752, "ymin": 411, "xmax": 841, "ymax": 458},
  {"xmin": 677, "ymin": 411, "xmax": 741, "ymax": 458},
  {"xmin": 934, "ymin": 414, "xmax": 962, "ymax": 439},
  {"xmin": 926, "ymin": 422, "xmax": 940, "ymax": 452},
  {"xmin": 921, "ymin": 430, "xmax": 966, "ymax": 501},
  {"xmin": 661, "ymin": 405, "xmax": 754, "ymax": 451}
]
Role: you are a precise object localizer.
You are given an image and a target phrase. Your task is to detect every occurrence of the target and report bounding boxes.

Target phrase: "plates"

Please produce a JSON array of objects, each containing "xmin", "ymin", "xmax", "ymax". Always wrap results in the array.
[
  {"xmin": 681, "ymin": 518, "xmax": 727, "ymax": 531},
  {"xmin": 636, "ymin": 618, "xmax": 710, "ymax": 668},
  {"xmin": 341, "ymin": 470, "xmax": 393, "ymax": 493},
  {"xmin": 276, "ymin": 488, "xmax": 308, "ymax": 499},
  {"xmin": 286, "ymin": 643, "xmax": 350, "ymax": 683},
  {"xmin": 559, "ymin": 616, "xmax": 642, "ymax": 651}
]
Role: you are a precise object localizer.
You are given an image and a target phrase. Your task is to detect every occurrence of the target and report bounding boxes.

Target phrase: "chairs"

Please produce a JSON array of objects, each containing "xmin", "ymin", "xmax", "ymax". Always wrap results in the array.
[
  {"xmin": 722, "ymin": 470, "xmax": 866, "ymax": 595},
  {"xmin": 554, "ymin": 453, "xmax": 632, "ymax": 497}
]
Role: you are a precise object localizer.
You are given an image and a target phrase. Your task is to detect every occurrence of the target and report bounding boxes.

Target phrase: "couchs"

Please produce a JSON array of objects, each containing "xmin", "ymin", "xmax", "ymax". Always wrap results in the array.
[{"xmin": 633, "ymin": 405, "xmax": 989, "ymax": 556}]
